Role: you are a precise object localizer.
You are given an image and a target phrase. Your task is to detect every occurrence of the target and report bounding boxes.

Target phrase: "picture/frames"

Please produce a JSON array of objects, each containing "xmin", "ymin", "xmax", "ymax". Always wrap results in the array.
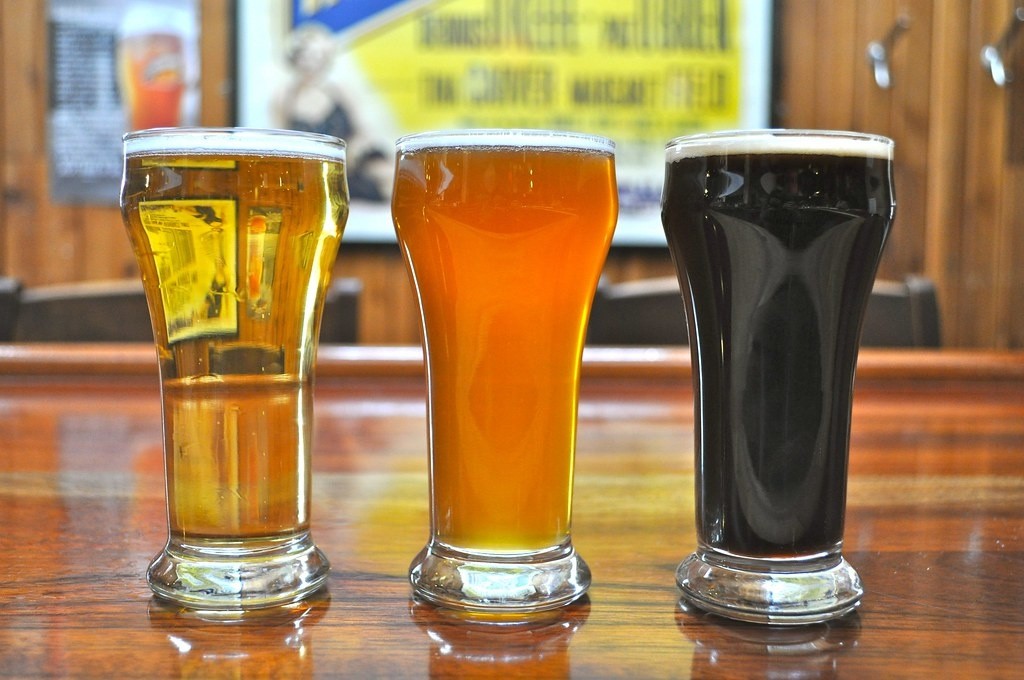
[{"xmin": 227, "ymin": 0, "xmax": 783, "ymax": 257}]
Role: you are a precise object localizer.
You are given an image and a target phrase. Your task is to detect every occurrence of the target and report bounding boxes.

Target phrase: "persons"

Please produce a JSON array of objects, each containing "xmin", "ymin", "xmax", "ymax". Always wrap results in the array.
[{"xmin": 269, "ymin": 22, "xmax": 382, "ymax": 201}]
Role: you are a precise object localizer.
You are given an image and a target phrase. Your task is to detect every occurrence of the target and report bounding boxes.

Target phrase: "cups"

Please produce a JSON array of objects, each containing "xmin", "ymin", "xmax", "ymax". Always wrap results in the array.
[
  {"xmin": 391, "ymin": 129, "xmax": 620, "ymax": 614},
  {"xmin": 663, "ymin": 131, "xmax": 896, "ymax": 625},
  {"xmin": 119, "ymin": 128, "xmax": 349, "ymax": 610}
]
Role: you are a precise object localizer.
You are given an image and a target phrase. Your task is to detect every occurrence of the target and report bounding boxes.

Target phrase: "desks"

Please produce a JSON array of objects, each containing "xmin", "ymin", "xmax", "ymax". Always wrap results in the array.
[{"xmin": 0, "ymin": 341, "xmax": 1024, "ymax": 680}]
